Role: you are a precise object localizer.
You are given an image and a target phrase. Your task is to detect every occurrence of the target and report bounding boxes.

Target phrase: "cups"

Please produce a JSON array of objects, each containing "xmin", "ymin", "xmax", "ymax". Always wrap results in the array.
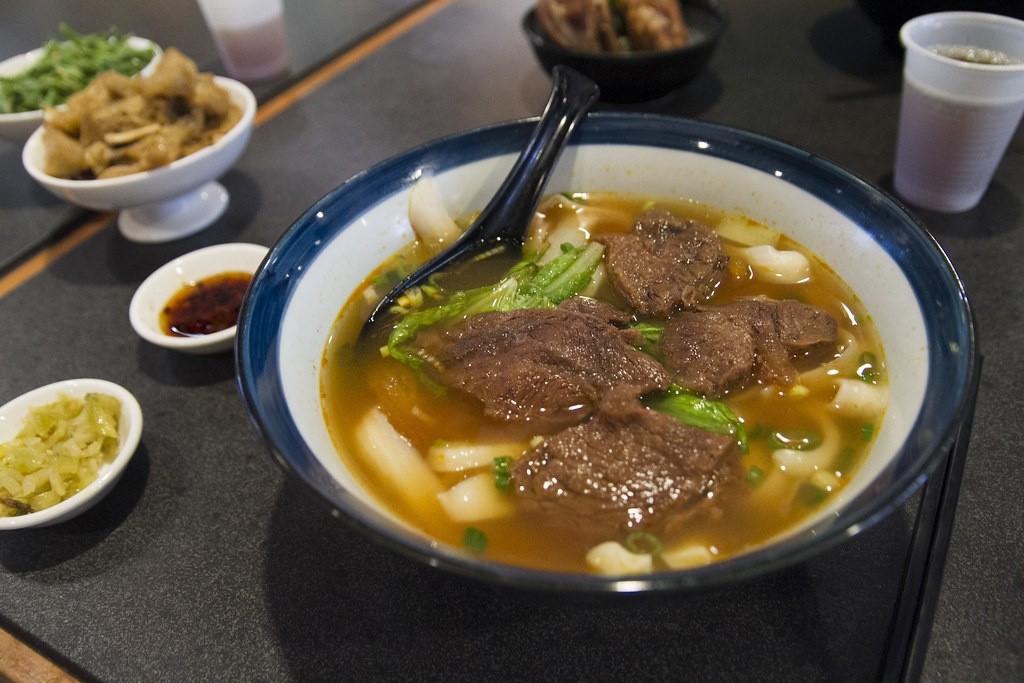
[
  {"xmin": 197, "ymin": 0, "xmax": 290, "ymax": 84},
  {"xmin": 893, "ymin": 11, "xmax": 1024, "ymax": 215}
]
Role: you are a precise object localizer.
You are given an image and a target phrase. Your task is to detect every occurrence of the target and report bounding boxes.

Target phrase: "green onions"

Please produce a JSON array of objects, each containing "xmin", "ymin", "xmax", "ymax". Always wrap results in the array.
[
  {"xmin": 335, "ymin": 253, "xmax": 417, "ymax": 375},
  {"xmin": 463, "ymin": 348, "xmax": 878, "ymax": 557}
]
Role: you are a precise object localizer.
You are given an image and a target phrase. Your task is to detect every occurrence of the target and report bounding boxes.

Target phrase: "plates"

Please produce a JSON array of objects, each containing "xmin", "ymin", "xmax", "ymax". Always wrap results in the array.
[
  {"xmin": 129, "ymin": 242, "xmax": 270, "ymax": 355},
  {"xmin": 0, "ymin": 379, "xmax": 143, "ymax": 530}
]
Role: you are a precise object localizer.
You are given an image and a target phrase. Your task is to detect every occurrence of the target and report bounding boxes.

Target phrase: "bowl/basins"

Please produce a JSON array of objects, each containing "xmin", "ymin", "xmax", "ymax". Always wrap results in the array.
[
  {"xmin": 0, "ymin": 36, "xmax": 164, "ymax": 143},
  {"xmin": 522, "ymin": 0, "xmax": 726, "ymax": 104},
  {"xmin": 234, "ymin": 113, "xmax": 974, "ymax": 593},
  {"xmin": 810, "ymin": 0, "xmax": 1024, "ymax": 92},
  {"xmin": 22, "ymin": 76, "xmax": 257, "ymax": 244}
]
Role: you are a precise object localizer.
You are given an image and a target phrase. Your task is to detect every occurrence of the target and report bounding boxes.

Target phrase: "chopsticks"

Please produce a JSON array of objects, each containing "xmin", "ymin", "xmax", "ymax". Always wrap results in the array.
[{"xmin": 877, "ymin": 351, "xmax": 982, "ymax": 683}]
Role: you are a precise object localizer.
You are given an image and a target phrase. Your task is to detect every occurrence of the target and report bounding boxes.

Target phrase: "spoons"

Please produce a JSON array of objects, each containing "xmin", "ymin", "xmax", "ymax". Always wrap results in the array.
[{"xmin": 355, "ymin": 65, "xmax": 601, "ymax": 357}]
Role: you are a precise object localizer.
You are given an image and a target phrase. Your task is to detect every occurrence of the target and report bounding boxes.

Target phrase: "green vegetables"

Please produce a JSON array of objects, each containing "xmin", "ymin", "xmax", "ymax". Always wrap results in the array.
[
  {"xmin": 376, "ymin": 238, "xmax": 751, "ymax": 457},
  {"xmin": 0, "ymin": 21, "xmax": 154, "ymax": 115}
]
